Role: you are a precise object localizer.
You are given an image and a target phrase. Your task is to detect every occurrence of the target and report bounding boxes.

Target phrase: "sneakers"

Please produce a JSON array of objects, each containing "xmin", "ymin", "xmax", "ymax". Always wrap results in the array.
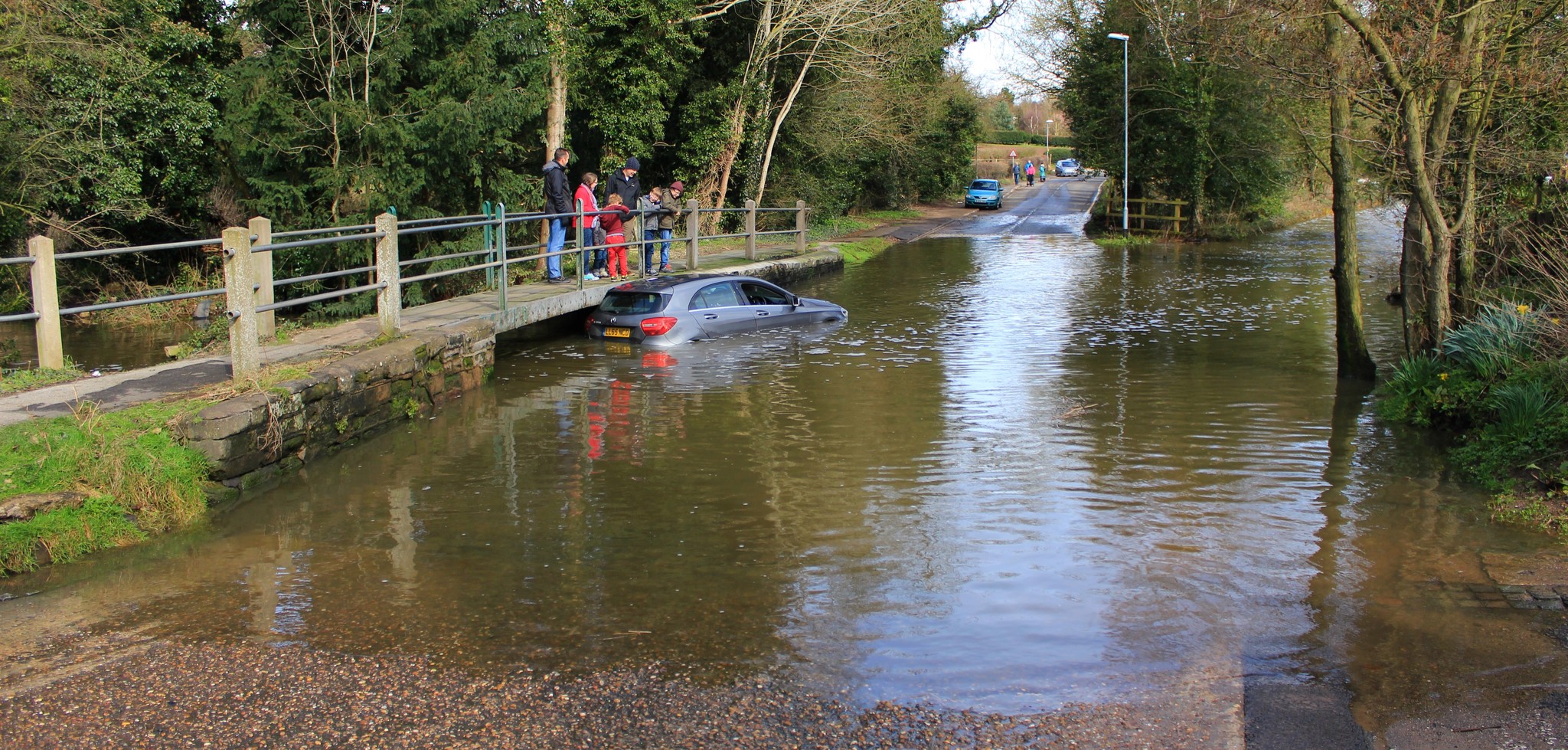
[
  {"xmin": 659, "ymin": 266, "xmax": 675, "ymax": 273},
  {"xmin": 550, "ymin": 275, "xmax": 571, "ymax": 284},
  {"xmin": 584, "ymin": 273, "xmax": 599, "ymax": 281},
  {"xmin": 645, "ymin": 268, "xmax": 659, "ymax": 276},
  {"xmin": 547, "ymin": 277, "xmax": 550, "ymax": 282}
]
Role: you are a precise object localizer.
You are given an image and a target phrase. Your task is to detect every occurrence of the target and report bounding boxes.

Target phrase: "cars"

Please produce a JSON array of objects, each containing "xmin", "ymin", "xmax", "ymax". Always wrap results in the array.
[
  {"xmin": 585, "ymin": 271, "xmax": 849, "ymax": 347},
  {"xmin": 963, "ymin": 179, "xmax": 1004, "ymax": 210},
  {"xmin": 1055, "ymin": 158, "xmax": 1080, "ymax": 177}
]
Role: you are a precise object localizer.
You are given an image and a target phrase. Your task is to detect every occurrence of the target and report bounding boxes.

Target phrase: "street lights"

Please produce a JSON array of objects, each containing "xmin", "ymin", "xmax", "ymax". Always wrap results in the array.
[
  {"xmin": 1046, "ymin": 120, "xmax": 1053, "ymax": 170},
  {"xmin": 1108, "ymin": 32, "xmax": 1130, "ymax": 238}
]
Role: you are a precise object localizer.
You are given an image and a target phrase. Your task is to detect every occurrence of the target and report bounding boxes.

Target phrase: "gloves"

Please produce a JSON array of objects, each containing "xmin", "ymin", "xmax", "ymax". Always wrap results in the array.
[{"xmin": 561, "ymin": 217, "xmax": 569, "ymax": 228}]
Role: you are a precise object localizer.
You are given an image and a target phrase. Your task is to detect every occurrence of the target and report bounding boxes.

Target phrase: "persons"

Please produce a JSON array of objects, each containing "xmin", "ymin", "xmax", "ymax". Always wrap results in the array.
[
  {"xmin": 642, "ymin": 187, "xmax": 663, "ymax": 276},
  {"xmin": 658, "ymin": 181, "xmax": 683, "ymax": 273},
  {"xmin": 571, "ymin": 172, "xmax": 600, "ymax": 281},
  {"xmin": 592, "ymin": 208, "xmax": 611, "ymax": 278},
  {"xmin": 604, "ymin": 157, "xmax": 640, "ymax": 275},
  {"xmin": 599, "ymin": 193, "xmax": 630, "ymax": 282},
  {"xmin": 542, "ymin": 148, "xmax": 574, "ymax": 283},
  {"xmin": 1013, "ymin": 163, "xmax": 1020, "ymax": 185},
  {"xmin": 1025, "ymin": 160, "xmax": 1035, "ymax": 186},
  {"xmin": 1038, "ymin": 163, "xmax": 1046, "ymax": 182}
]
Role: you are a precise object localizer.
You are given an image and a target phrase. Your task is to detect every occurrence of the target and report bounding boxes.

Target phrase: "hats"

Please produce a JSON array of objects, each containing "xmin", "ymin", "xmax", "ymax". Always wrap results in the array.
[
  {"xmin": 670, "ymin": 181, "xmax": 683, "ymax": 196},
  {"xmin": 625, "ymin": 156, "xmax": 640, "ymax": 170}
]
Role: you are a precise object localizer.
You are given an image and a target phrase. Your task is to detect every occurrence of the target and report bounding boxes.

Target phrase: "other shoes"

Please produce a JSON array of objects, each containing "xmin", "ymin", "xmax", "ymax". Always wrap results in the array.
[
  {"xmin": 600, "ymin": 270, "xmax": 610, "ymax": 278},
  {"xmin": 618, "ymin": 270, "xmax": 635, "ymax": 276},
  {"xmin": 611, "ymin": 276, "xmax": 621, "ymax": 282},
  {"xmin": 621, "ymin": 274, "xmax": 629, "ymax": 281},
  {"xmin": 593, "ymin": 272, "xmax": 601, "ymax": 279}
]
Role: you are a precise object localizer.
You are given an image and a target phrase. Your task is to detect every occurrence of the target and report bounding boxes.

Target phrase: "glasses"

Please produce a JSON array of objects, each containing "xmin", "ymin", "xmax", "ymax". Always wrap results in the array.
[
  {"xmin": 673, "ymin": 188, "xmax": 681, "ymax": 196},
  {"xmin": 650, "ymin": 194, "xmax": 658, "ymax": 200},
  {"xmin": 593, "ymin": 182, "xmax": 598, "ymax": 186}
]
